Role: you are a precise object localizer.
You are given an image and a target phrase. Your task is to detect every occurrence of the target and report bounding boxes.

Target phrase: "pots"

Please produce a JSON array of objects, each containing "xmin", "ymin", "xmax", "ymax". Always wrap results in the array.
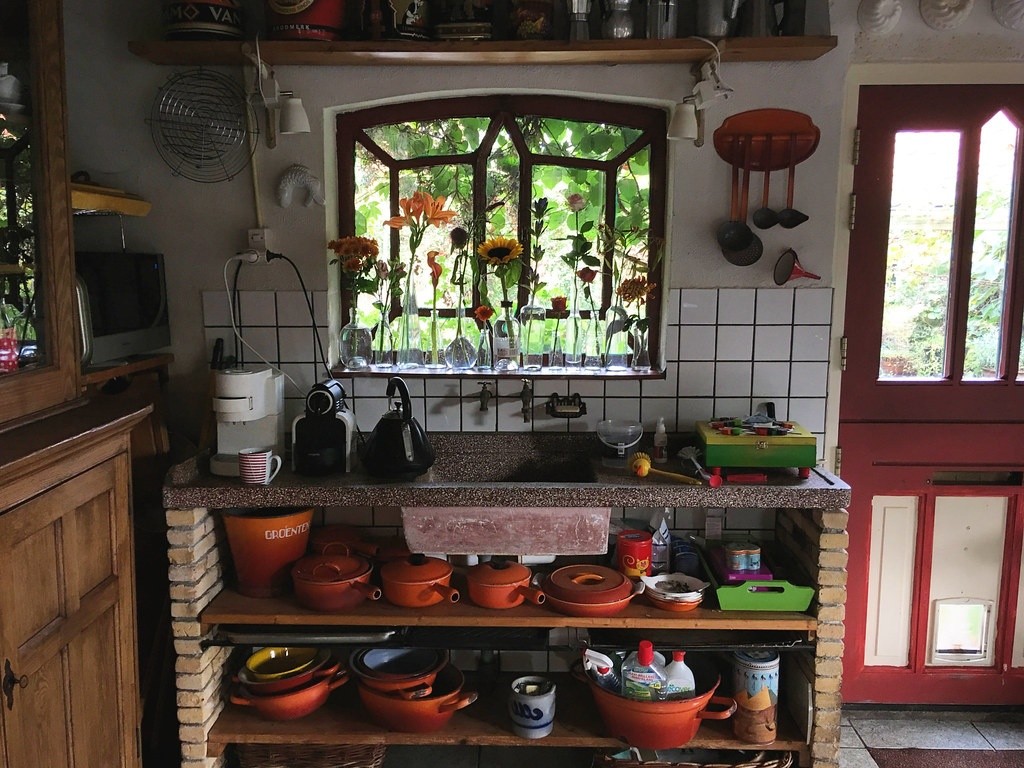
[
  {"xmin": 290, "ymin": 522, "xmax": 645, "ymax": 616},
  {"xmin": 229, "ymin": 647, "xmax": 480, "ymax": 735},
  {"xmin": 570, "ymin": 657, "xmax": 737, "ymax": 751}
]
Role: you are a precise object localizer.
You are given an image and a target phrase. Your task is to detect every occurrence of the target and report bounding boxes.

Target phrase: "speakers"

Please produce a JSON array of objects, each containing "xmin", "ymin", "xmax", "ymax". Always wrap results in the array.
[{"xmin": 291, "ymin": 407, "xmax": 355, "ymax": 477}]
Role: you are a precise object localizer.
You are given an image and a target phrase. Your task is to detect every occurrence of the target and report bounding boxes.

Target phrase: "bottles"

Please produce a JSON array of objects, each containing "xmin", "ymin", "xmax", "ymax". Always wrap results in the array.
[
  {"xmin": 263, "ymin": 0, "xmax": 346, "ymax": 43},
  {"xmin": 581, "ymin": 640, "xmax": 668, "ymax": 703},
  {"xmin": 432, "ymin": 0, "xmax": 495, "ymax": 41},
  {"xmin": 732, "ymin": 648, "xmax": 780, "ymax": 746},
  {"xmin": 353, "ymin": 0, "xmax": 432, "ymax": 42},
  {"xmin": 506, "ymin": 0, "xmax": 554, "ymax": 40},
  {"xmin": 663, "ymin": 651, "xmax": 696, "ymax": 701},
  {"xmin": 160, "ymin": 0, "xmax": 246, "ymax": 42},
  {"xmin": 338, "ymin": 300, "xmax": 651, "ymax": 371}
]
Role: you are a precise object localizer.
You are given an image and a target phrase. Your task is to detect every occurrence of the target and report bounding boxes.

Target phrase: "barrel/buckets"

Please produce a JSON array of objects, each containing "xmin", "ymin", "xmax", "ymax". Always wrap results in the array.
[
  {"xmin": 221, "ymin": 507, "xmax": 314, "ymax": 599},
  {"xmin": 596, "ymin": 419, "xmax": 643, "ymax": 469}
]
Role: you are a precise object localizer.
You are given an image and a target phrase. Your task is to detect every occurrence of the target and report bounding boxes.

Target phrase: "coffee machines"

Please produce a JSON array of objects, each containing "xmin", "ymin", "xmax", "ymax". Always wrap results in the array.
[{"xmin": 209, "ymin": 366, "xmax": 286, "ymax": 478}]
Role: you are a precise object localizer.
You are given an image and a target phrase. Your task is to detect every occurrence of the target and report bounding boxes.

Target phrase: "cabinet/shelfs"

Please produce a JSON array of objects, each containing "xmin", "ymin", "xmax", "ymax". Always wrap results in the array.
[
  {"xmin": 0, "ymin": 393, "xmax": 156, "ymax": 768},
  {"xmin": 0, "ymin": 0, "xmax": 90, "ymax": 434},
  {"xmin": 161, "ymin": 433, "xmax": 851, "ymax": 768}
]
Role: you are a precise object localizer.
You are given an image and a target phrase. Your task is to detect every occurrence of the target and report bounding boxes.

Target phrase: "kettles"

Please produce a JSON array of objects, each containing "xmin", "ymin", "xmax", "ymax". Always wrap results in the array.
[{"xmin": 362, "ymin": 376, "xmax": 438, "ymax": 478}]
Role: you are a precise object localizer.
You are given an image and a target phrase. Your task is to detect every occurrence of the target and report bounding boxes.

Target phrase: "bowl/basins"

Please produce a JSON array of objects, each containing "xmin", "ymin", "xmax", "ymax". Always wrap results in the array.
[
  {"xmin": 638, "ymin": 572, "xmax": 711, "ymax": 612},
  {"xmin": 0, "ymin": 75, "xmax": 24, "ymax": 104}
]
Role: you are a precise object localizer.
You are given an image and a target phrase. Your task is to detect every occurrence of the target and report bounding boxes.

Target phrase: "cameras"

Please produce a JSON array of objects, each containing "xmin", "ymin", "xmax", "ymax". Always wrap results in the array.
[{"xmin": 302, "ymin": 378, "xmax": 347, "ymax": 418}]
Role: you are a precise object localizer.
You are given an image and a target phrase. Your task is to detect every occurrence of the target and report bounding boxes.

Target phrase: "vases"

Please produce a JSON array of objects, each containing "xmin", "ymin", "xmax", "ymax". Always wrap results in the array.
[
  {"xmin": 604, "ymin": 315, "xmax": 628, "ymax": 370},
  {"xmin": 338, "ymin": 308, "xmax": 373, "ymax": 370},
  {"xmin": 425, "ymin": 311, "xmax": 444, "ymax": 369},
  {"xmin": 398, "ymin": 294, "xmax": 424, "ymax": 368},
  {"xmin": 374, "ymin": 317, "xmax": 395, "ymax": 367},
  {"xmin": 493, "ymin": 313, "xmax": 520, "ymax": 357},
  {"xmin": 548, "ymin": 326, "xmax": 566, "ymax": 368},
  {"xmin": 445, "ymin": 307, "xmax": 478, "ymax": 369},
  {"xmin": 520, "ymin": 304, "xmax": 548, "ymax": 371},
  {"xmin": 584, "ymin": 312, "xmax": 603, "ymax": 369},
  {"xmin": 564, "ymin": 308, "xmax": 585, "ymax": 367},
  {"xmin": 477, "ymin": 328, "xmax": 492, "ymax": 369},
  {"xmin": 631, "ymin": 325, "xmax": 653, "ymax": 372}
]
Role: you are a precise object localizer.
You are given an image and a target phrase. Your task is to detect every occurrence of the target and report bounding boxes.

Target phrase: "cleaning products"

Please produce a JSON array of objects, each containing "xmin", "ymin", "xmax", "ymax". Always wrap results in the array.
[{"xmin": 579, "ymin": 639, "xmax": 695, "ymax": 702}]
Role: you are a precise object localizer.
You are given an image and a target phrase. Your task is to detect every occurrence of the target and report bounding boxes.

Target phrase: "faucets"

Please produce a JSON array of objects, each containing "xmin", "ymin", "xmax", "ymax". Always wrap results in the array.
[
  {"xmin": 477, "ymin": 381, "xmax": 494, "ymax": 411},
  {"xmin": 520, "ymin": 378, "xmax": 533, "ymax": 423}
]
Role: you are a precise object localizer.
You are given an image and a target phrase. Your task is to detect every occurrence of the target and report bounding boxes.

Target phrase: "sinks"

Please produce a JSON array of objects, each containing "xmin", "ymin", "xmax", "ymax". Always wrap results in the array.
[{"xmin": 412, "ymin": 431, "xmax": 599, "ymax": 484}]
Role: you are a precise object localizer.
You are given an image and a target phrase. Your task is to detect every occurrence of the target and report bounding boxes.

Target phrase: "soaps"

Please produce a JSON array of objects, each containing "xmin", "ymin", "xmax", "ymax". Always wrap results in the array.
[{"xmin": 555, "ymin": 405, "xmax": 580, "ymax": 412}]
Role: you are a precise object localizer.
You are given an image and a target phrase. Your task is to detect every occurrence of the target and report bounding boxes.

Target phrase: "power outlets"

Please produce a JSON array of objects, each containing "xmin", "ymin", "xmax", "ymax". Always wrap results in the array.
[{"xmin": 248, "ymin": 229, "xmax": 272, "ymax": 265}]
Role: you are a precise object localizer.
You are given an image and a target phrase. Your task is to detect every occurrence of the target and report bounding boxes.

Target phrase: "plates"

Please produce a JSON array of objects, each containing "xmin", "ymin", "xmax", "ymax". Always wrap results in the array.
[{"xmin": 0, "ymin": 102, "xmax": 27, "ymax": 114}]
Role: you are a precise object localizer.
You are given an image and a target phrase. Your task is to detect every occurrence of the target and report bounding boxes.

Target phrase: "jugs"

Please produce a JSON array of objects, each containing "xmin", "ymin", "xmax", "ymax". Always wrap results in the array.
[
  {"xmin": 598, "ymin": 0, "xmax": 636, "ymax": 39},
  {"xmin": 739, "ymin": 0, "xmax": 789, "ymax": 40},
  {"xmin": 642, "ymin": 0, "xmax": 678, "ymax": 39},
  {"xmin": 694, "ymin": 0, "xmax": 745, "ymax": 38}
]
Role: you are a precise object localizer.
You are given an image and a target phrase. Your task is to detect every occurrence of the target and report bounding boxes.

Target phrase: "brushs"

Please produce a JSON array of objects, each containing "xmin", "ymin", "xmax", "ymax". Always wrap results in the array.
[
  {"xmin": 677, "ymin": 447, "xmax": 711, "ymax": 481},
  {"xmin": 628, "ymin": 452, "xmax": 702, "ymax": 484}
]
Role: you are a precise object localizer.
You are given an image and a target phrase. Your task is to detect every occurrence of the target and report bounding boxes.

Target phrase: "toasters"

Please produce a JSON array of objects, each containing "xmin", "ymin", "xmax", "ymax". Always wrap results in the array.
[{"xmin": 291, "ymin": 377, "xmax": 356, "ymax": 474}]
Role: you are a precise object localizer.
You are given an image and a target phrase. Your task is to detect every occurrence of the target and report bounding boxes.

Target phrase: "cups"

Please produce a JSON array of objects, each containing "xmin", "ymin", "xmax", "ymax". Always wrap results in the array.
[
  {"xmin": 508, "ymin": 675, "xmax": 556, "ymax": 739},
  {"xmin": 279, "ymin": 97, "xmax": 311, "ymax": 135},
  {"xmin": 238, "ymin": 447, "xmax": 282, "ymax": 486}
]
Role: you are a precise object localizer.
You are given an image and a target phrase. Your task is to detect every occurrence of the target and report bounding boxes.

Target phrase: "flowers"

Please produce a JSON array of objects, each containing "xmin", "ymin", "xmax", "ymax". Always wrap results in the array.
[{"xmin": 327, "ymin": 190, "xmax": 665, "ymax": 367}]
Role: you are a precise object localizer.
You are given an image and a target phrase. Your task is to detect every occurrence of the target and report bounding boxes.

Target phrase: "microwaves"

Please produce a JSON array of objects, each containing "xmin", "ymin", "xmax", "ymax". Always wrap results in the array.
[{"xmin": 74, "ymin": 252, "xmax": 171, "ymax": 369}]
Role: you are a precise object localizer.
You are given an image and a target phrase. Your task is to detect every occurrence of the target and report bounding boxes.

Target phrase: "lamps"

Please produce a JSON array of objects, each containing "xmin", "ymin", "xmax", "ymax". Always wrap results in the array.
[
  {"xmin": 667, "ymin": 62, "xmax": 733, "ymax": 140},
  {"xmin": 245, "ymin": 67, "xmax": 310, "ymax": 135}
]
